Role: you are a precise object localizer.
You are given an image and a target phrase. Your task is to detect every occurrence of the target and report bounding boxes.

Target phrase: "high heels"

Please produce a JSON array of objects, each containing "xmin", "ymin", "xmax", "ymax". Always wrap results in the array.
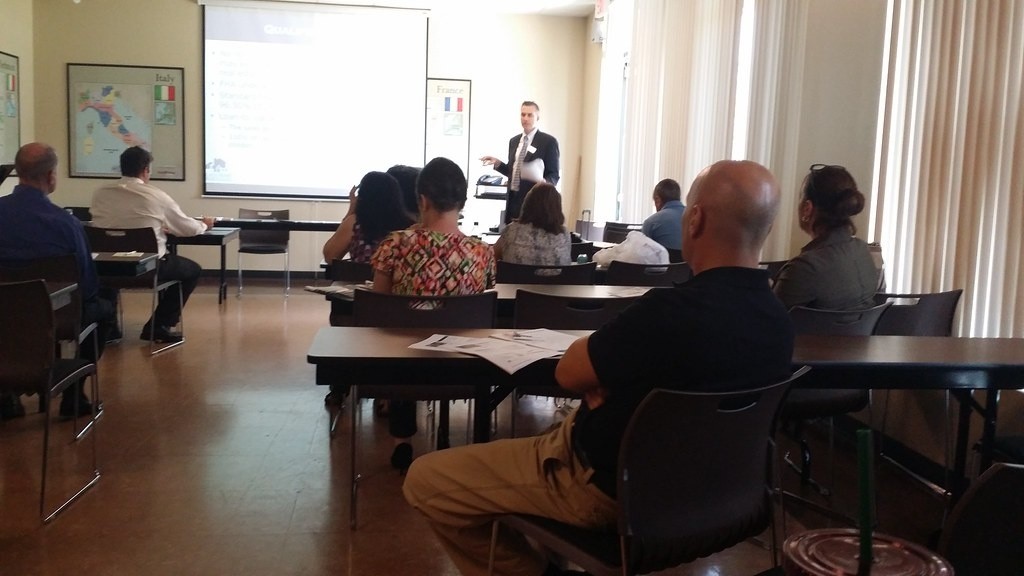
[{"xmin": 390, "ymin": 441, "xmax": 413, "ymax": 477}]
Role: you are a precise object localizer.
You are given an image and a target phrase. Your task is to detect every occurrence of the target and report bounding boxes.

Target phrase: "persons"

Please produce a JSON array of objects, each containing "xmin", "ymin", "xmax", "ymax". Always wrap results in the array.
[
  {"xmin": 771, "ymin": 164, "xmax": 880, "ymax": 411},
  {"xmin": 494, "ymin": 183, "xmax": 572, "ymax": 276},
  {"xmin": 642, "ymin": 178, "xmax": 686, "ymax": 251},
  {"xmin": 478, "ymin": 101, "xmax": 560, "ymax": 225},
  {"xmin": 370, "ymin": 157, "xmax": 496, "ymax": 311},
  {"xmin": 322, "ymin": 164, "xmax": 421, "ymax": 264},
  {"xmin": 87, "ymin": 146, "xmax": 214, "ymax": 344},
  {"xmin": 404, "ymin": 159, "xmax": 794, "ymax": 576},
  {"xmin": 0, "ymin": 143, "xmax": 103, "ymax": 419}
]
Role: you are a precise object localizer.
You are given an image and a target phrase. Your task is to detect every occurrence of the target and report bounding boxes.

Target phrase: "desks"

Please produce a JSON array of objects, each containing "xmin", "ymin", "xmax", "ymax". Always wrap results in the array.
[
  {"xmin": 165, "ymin": 229, "xmax": 240, "ymax": 303},
  {"xmin": 306, "ymin": 327, "xmax": 1024, "ymax": 527},
  {"xmin": 327, "ymin": 281, "xmax": 674, "ymax": 328},
  {"xmin": 194, "ymin": 217, "xmax": 342, "ymax": 231},
  {"xmin": 0, "ymin": 275, "xmax": 79, "ymax": 310},
  {"xmin": 88, "ymin": 250, "xmax": 158, "ymax": 280}
]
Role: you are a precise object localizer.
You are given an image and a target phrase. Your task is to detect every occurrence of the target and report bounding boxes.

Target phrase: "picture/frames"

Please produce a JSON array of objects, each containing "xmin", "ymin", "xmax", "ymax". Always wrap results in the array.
[
  {"xmin": 426, "ymin": 78, "xmax": 472, "ymax": 188},
  {"xmin": 0, "ymin": 51, "xmax": 21, "ymax": 167},
  {"xmin": 67, "ymin": 62, "xmax": 186, "ymax": 181}
]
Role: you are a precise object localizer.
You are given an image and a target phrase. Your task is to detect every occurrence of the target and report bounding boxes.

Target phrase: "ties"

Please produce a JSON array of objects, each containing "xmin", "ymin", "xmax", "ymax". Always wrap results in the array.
[{"xmin": 509, "ymin": 135, "xmax": 529, "ymax": 193}]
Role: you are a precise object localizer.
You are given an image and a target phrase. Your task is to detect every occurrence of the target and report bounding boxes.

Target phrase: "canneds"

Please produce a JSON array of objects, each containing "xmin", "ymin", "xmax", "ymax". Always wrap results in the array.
[{"xmin": 577, "ymin": 254, "xmax": 588, "ymax": 264}]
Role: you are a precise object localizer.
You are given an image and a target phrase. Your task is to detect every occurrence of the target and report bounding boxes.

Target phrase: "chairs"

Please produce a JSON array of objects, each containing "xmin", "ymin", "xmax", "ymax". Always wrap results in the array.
[
  {"xmin": 80, "ymin": 226, "xmax": 186, "ymax": 354},
  {"xmin": 237, "ymin": 208, "xmax": 291, "ymax": 297},
  {"xmin": 329, "ymin": 218, "xmax": 1024, "ymax": 576},
  {"xmin": 1, "ymin": 253, "xmax": 105, "ymax": 524}
]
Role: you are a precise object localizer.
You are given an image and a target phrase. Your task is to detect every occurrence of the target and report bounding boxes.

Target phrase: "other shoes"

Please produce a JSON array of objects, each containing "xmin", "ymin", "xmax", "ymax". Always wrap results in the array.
[
  {"xmin": 140, "ymin": 318, "xmax": 183, "ymax": 342},
  {"xmin": 59, "ymin": 397, "xmax": 104, "ymax": 417},
  {"xmin": 373, "ymin": 397, "xmax": 392, "ymax": 418},
  {"xmin": 324, "ymin": 390, "xmax": 358, "ymax": 407}
]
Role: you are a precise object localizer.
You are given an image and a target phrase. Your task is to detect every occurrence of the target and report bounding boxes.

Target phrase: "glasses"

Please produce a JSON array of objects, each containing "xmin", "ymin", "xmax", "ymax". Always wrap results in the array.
[{"xmin": 808, "ymin": 163, "xmax": 846, "ymax": 194}]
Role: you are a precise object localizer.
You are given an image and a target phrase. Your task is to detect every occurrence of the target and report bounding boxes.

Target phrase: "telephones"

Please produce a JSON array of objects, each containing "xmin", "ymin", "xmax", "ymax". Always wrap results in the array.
[{"xmin": 478, "ymin": 174, "xmax": 503, "ymax": 185}]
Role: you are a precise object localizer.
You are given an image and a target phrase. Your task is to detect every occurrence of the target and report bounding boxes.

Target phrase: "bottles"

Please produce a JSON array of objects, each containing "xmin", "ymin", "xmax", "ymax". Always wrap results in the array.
[{"xmin": 471, "ymin": 221, "xmax": 483, "ymax": 240}]
